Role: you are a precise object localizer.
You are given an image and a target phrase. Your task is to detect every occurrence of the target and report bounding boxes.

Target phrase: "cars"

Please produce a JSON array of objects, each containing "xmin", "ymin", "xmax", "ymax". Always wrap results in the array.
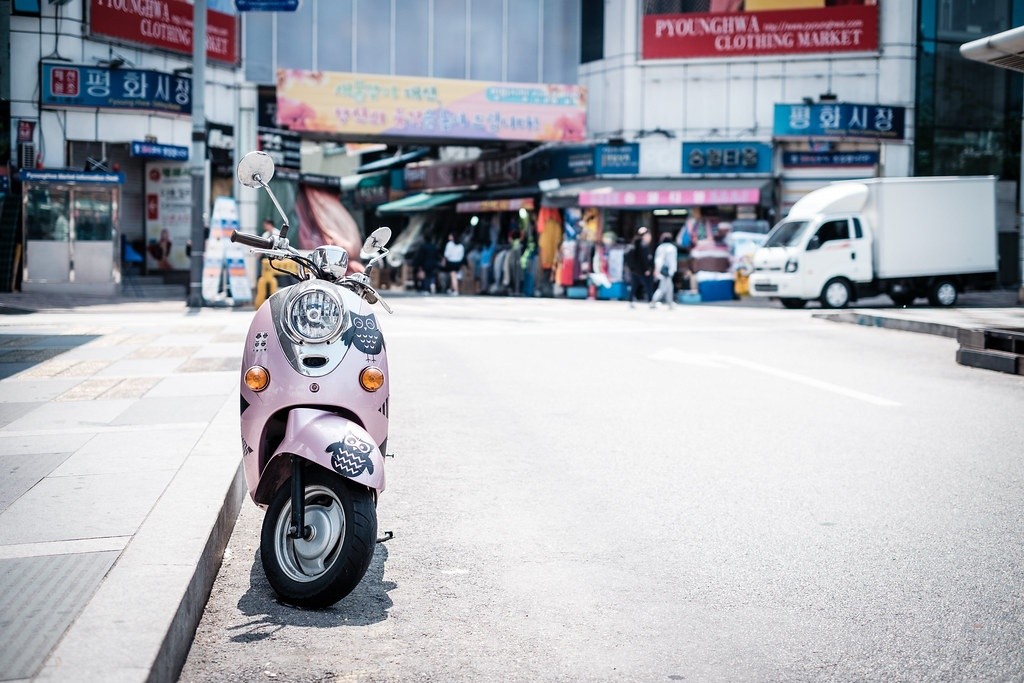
[{"xmin": 728, "ymin": 220, "xmax": 769, "ymax": 253}]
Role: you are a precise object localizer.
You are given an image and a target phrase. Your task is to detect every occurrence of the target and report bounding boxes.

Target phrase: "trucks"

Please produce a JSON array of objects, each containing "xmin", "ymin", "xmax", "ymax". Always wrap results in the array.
[{"xmin": 750, "ymin": 174, "xmax": 996, "ymax": 314}]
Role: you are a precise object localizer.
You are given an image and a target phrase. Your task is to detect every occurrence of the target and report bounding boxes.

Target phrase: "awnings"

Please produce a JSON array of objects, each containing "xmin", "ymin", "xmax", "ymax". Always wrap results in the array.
[{"xmin": 378, "ymin": 177, "xmax": 776, "ymax": 212}]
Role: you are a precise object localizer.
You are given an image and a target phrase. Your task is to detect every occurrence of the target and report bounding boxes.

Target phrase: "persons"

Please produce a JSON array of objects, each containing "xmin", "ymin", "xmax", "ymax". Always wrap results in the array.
[
  {"xmin": 50, "ymin": 203, "xmax": 74, "ymax": 240},
  {"xmin": 390, "ymin": 226, "xmax": 678, "ymax": 310},
  {"xmin": 261, "ymin": 218, "xmax": 281, "ymax": 257}
]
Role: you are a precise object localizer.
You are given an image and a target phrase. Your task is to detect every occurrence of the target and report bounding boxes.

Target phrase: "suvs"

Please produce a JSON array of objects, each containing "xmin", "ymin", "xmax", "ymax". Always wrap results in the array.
[{"xmin": 225, "ymin": 153, "xmax": 405, "ymax": 616}]
